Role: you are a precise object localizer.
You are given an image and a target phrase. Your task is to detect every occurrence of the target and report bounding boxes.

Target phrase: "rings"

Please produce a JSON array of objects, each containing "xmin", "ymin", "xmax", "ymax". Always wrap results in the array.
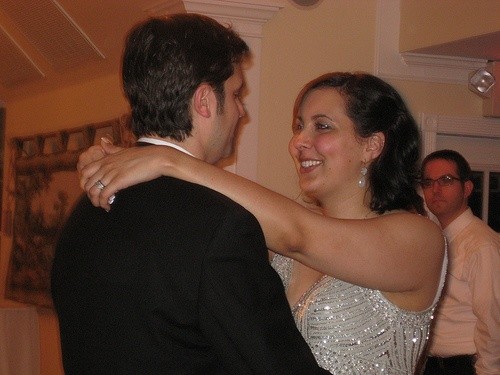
[{"xmin": 95, "ymin": 179, "xmax": 106, "ymax": 191}]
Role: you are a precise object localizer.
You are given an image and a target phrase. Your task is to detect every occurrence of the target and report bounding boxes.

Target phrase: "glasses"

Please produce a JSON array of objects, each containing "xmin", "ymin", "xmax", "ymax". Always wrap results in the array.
[{"xmin": 421, "ymin": 175, "xmax": 464, "ymax": 188}]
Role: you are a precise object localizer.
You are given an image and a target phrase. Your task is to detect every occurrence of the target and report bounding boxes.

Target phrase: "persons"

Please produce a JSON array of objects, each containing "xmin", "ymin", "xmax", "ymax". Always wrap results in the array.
[
  {"xmin": 421, "ymin": 149, "xmax": 500, "ymax": 375},
  {"xmin": 49, "ymin": 12, "xmax": 333, "ymax": 375},
  {"xmin": 78, "ymin": 71, "xmax": 448, "ymax": 375}
]
